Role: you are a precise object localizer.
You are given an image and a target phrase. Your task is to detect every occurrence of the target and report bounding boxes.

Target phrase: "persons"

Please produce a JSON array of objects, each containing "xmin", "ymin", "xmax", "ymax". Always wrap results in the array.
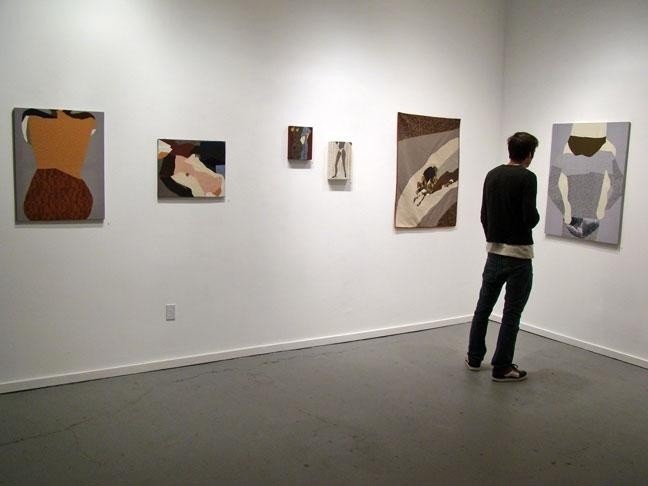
[
  {"xmin": 463, "ymin": 130, "xmax": 541, "ymax": 383},
  {"xmin": 331, "ymin": 142, "xmax": 347, "ymax": 178}
]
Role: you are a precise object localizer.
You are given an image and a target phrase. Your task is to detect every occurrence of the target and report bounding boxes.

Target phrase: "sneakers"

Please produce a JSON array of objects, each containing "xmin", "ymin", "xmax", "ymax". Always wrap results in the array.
[
  {"xmin": 465, "ymin": 351, "xmax": 481, "ymax": 370},
  {"xmin": 492, "ymin": 364, "xmax": 528, "ymax": 382}
]
